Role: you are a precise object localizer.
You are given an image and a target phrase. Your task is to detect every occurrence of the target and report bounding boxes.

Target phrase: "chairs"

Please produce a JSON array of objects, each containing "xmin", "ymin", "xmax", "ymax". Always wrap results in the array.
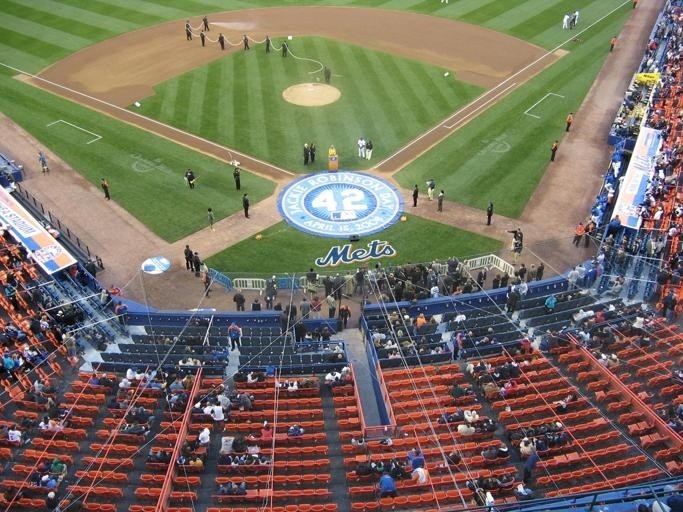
[
  {"xmin": 120, "ymin": 287, "xmax": 608, "ymax": 511},
  {"xmin": 2, "ymin": 230, "xmax": 120, "ymax": 510},
  {"xmin": 608, "ymin": 61, "xmax": 683, "ymax": 512}
]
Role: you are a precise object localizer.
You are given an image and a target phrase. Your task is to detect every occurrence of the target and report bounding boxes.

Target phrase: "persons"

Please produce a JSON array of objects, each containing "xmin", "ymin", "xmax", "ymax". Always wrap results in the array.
[
  {"xmin": 241, "ymin": 193, "xmax": 251, "ymax": 219},
  {"xmin": 365, "ymin": 140, "xmax": 373, "ymax": 162},
  {"xmin": 549, "ymin": 139, "xmax": 558, "ymax": 163},
  {"xmin": 185, "ymin": 169, "xmax": 195, "ymax": 191},
  {"xmin": 568, "ymin": 0, "xmax": 682, "ymax": 226},
  {"xmin": 426, "ymin": 179, "xmax": 435, "ymax": 201},
  {"xmin": 99, "ymin": 177, "xmax": 111, "ymax": 202},
  {"xmin": 308, "ymin": 141, "xmax": 316, "ymax": 164},
  {"xmin": 356, "ymin": 135, "xmax": 366, "ymax": 160},
  {"xmin": 435, "ymin": 189, "xmax": 445, "ymax": 213},
  {"xmin": 232, "ymin": 166, "xmax": 242, "ymax": 192},
  {"xmin": 485, "ymin": 201, "xmax": 494, "ymax": 226},
  {"xmin": 183, "ymin": 15, "xmax": 288, "ymax": 59},
  {"xmin": 207, "ymin": 206, "xmax": 216, "ymax": 234},
  {"xmin": 323, "ymin": 64, "xmax": 331, "ymax": 85},
  {"xmin": 561, "ymin": 11, "xmax": 570, "ymax": 30},
  {"xmin": 410, "ymin": 184, "xmax": 419, "ymax": 208},
  {"xmin": 327, "ymin": 145, "xmax": 337, "ymax": 157},
  {"xmin": 564, "ymin": 112, "xmax": 574, "ymax": 132},
  {"xmin": 1, "ymin": 227, "xmax": 683, "ymax": 511},
  {"xmin": 302, "ymin": 143, "xmax": 310, "ymax": 165},
  {"xmin": 37, "ymin": 152, "xmax": 49, "ymax": 175}
]
